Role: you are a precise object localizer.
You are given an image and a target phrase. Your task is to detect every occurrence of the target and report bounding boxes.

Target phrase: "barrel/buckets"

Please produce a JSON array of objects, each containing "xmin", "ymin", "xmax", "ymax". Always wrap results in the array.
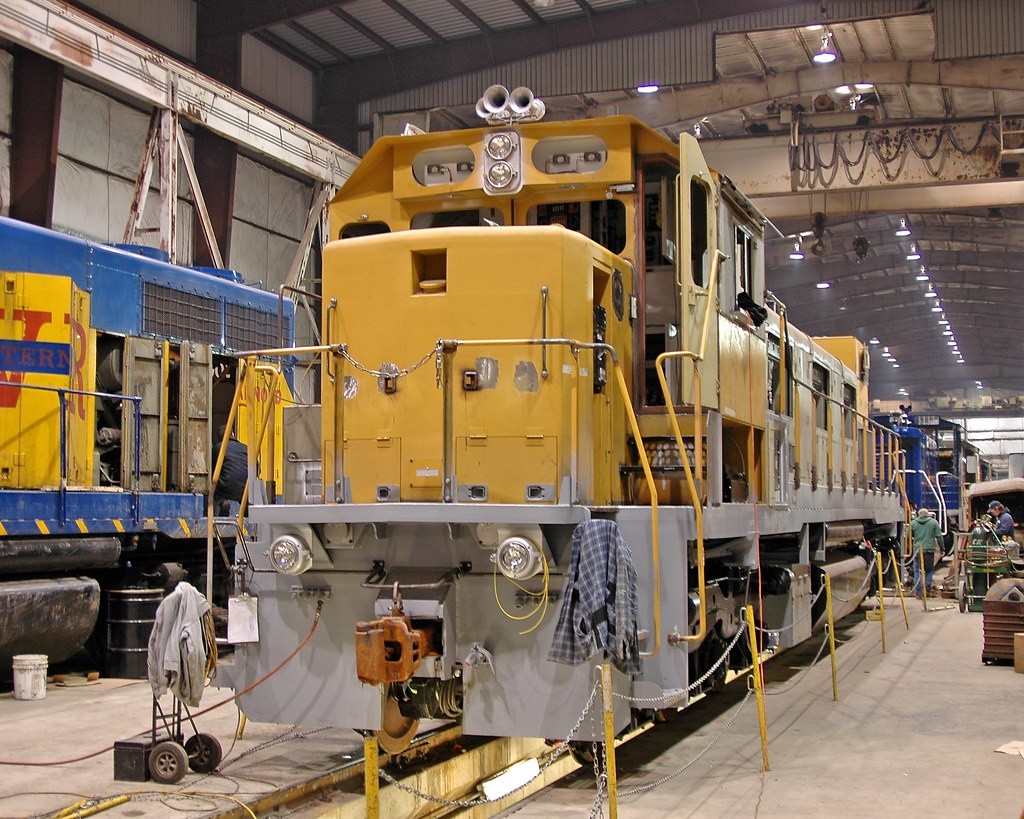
[
  {"xmin": 107, "ymin": 589, "xmax": 165, "ymax": 678},
  {"xmin": 982, "ymin": 601, "xmax": 1024, "ymax": 660},
  {"xmin": 13, "ymin": 654, "xmax": 48, "ymax": 700}
]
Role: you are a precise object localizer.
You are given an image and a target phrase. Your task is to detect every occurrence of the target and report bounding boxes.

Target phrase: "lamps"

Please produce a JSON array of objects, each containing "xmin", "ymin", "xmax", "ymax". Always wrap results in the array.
[
  {"xmin": 636, "ymin": 24, "xmax": 875, "ymax": 140},
  {"xmin": 787, "ymin": 214, "xmax": 984, "ymax": 396}
]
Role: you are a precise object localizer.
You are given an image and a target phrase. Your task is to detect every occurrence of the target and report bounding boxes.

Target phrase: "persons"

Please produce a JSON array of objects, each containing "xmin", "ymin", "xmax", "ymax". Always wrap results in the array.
[
  {"xmin": 988, "ymin": 500, "xmax": 1015, "ymax": 543},
  {"xmin": 911, "ymin": 507, "xmax": 945, "ymax": 600},
  {"xmin": 212, "ymin": 424, "xmax": 249, "ymax": 518}
]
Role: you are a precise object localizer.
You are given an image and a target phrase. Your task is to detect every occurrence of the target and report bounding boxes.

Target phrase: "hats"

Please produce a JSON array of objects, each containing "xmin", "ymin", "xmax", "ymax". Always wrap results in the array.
[
  {"xmin": 987, "ymin": 501, "xmax": 1000, "ymax": 513},
  {"xmin": 919, "ymin": 509, "xmax": 928, "ymax": 517}
]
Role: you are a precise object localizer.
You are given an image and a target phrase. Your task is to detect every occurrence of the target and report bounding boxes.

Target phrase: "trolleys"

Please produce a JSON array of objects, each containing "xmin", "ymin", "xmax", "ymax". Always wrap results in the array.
[{"xmin": 148, "ymin": 694, "xmax": 222, "ymax": 785}]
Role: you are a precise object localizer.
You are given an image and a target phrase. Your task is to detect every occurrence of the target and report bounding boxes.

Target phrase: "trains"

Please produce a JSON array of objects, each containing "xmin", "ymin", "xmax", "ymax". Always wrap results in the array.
[{"xmin": 205, "ymin": 85, "xmax": 912, "ymax": 765}]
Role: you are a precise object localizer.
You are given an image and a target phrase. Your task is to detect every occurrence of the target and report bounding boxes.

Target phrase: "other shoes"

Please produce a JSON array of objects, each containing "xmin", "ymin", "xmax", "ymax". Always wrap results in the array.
[{"xmin": 219, "ymin": 500, "xmax": 230, "ymax": 517}]
